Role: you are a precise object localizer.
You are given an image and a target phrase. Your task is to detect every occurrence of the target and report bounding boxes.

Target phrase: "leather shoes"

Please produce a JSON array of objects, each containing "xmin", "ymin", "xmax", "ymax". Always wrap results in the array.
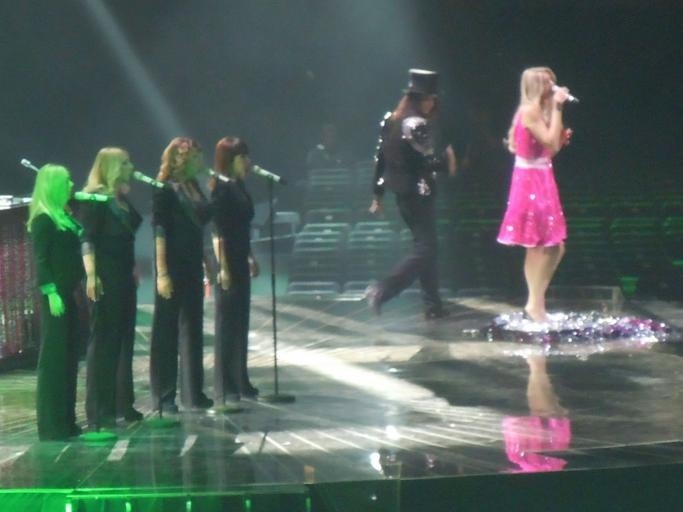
[
  {"xmin": 361, "ymin": 284, "xmax": 381, "ymax": 314},
  {"xmin": 40, "ymin": 383, "xmax": 259, "ymax": 442},
  {"xmin": 424, "ymin": 306, "xmax": 450, "ymax": 319}
]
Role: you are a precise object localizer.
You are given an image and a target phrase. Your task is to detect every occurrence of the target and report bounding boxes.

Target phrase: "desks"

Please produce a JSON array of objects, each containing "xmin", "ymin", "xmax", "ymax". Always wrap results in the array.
[{"xmin": 0, "ymin": 197, "xmax": 37, "ymax": 367}]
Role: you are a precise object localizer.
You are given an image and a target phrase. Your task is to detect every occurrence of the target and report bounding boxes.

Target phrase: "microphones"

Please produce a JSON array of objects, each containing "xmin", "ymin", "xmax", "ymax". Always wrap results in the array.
[
  {"xmin": 204, "ymin": 166, "xmax": 233, "ymax": 184},
  {"xmin": 551, "ymin": 85, "xmax": 579, "ymax": 106},
  {"xmin": 74, "ymin": 191, "xmax": 112, "ymax": 203},
  {"xmin": 252, "ymin": 165, "xmax": 288, "ymax": 185},
  {"xmin": 133, "ymin": 170, "xmax": 171, "ymax": 192},
  {"xmin": 21, "ymin": 158, "xmax": 38, "ymax": 172}
]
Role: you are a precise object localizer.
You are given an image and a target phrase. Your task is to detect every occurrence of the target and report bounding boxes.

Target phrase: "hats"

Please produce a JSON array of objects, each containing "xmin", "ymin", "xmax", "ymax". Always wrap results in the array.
[{"xmin": 403, "ymin": 68, "xmax": 441, "ymax": 97}]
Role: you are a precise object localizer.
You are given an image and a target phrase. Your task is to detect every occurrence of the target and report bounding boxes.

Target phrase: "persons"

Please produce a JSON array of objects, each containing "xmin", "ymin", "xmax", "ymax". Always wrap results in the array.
[
  {"xmin": 78, "ymin": 147, "xmax": 144, "ymax": 426},
  {"xmin": 23, "ymin": 164, "xmax": 88, "ymax": 440},
  {"xmin": 150, "ymin": 137, "xmax": 219, "ymax": 419},
  {"xmin": 503, "ymin": 354, "xmax": 572, "ymax": 473},
  {"xmin": 208, "ymin": 136, "xmax": 260, "ymax": 403},
  {"xmin": 363, "ymin": 68, "xmax": 458, "ymax": 322},
  {"xmin": 497, "ymin": 66, "xmax": 573, "ymax": 324}
]
